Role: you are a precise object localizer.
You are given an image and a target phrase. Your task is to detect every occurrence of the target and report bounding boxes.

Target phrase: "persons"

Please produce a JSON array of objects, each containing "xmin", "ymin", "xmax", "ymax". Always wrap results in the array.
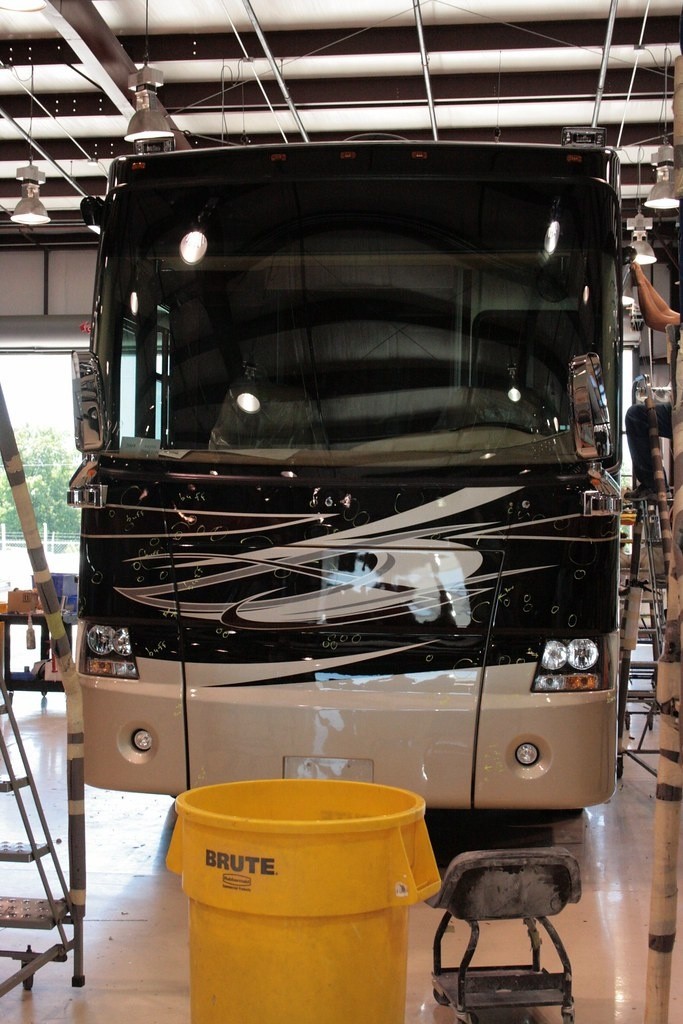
[{"xmin": 624, "ymin": 262, "xmax": 683, "ymax": 501}]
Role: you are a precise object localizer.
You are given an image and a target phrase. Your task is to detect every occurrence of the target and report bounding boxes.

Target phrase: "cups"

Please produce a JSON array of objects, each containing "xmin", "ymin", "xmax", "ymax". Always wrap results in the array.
[{"xmin": 0, "ymin": 601, "xmax": 8, "ymax": 614}]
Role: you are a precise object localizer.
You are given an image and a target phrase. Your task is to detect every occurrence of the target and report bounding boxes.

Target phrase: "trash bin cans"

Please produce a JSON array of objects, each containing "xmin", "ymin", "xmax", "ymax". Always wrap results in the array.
[{"xmin": 163, "ymin": 773, "xmax": 440, "ymax": 1024}]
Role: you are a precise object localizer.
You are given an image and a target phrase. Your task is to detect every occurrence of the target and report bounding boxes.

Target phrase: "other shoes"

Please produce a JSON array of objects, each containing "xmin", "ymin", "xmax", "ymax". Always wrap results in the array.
[{"xmin": 623, "ymin": 484, "xmax": 671, "ymax": 501}]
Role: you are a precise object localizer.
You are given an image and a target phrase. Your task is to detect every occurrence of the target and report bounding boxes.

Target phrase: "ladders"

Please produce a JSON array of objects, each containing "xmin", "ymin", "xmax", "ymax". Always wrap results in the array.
[{"xmin": 619, "ymin": 495, "xmax": 669, "ymax": 781}]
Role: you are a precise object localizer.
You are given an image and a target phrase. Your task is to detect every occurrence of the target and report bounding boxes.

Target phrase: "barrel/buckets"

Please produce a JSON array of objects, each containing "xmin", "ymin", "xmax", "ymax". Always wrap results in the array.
[{"xmin": 166, "ymin": 779, "xmax": 442, "ymax": 1024}]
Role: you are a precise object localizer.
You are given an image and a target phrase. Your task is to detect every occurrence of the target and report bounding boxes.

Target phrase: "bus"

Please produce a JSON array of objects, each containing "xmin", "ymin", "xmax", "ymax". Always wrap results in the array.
[{"xmin": 61, "ymin": 130, "xmax": 635, "ymax": 824}]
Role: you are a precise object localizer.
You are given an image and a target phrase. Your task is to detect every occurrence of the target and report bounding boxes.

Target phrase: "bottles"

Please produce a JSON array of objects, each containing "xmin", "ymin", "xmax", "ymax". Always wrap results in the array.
[{"xmin": 26, "ymin": 620, "xmax": 35, "ymax": 649}]
